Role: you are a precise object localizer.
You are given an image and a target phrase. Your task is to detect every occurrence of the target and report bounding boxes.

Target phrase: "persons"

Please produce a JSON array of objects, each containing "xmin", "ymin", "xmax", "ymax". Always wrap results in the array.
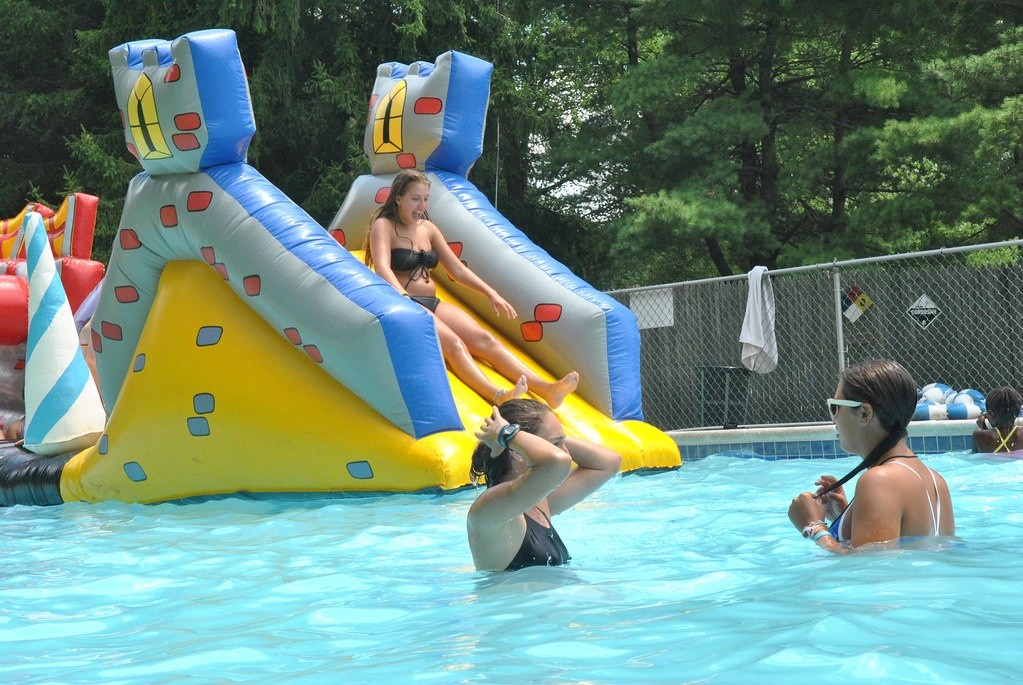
[
  {"xmin": 787, "ymin": 358, "xmax": 956, "ymax": 556},
  {"xmin": 466, "ymin": 398, "xmax": 621, "ymax": 574},
  {"xmin": 1, "ymin": 412, "xmax": 26, "ymax": 441},
  {"xmin": 363, "ymin": 168, "xmax": 580, "ymax": 410},
  {"xmin": 971, "ymin": 386, "xmax": 1023, "ymax": 454}
]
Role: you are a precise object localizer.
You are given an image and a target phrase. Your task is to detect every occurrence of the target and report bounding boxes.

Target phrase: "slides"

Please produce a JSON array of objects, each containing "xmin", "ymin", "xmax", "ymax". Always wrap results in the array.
[{"xmin": 190, "ymin": 161, "xmax": 683, "ymax": 496}]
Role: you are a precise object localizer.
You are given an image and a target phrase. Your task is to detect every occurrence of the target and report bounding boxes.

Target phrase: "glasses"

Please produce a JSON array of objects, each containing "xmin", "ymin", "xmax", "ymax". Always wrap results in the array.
[{"xmin": 827, "ymin": 397, "xmax": 863, "ymax": 417}]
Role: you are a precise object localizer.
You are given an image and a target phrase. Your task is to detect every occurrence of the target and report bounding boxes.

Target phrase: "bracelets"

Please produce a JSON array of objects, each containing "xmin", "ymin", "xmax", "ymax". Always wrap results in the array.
[
  {"xmin": 498, "ymin": 425, "xmax": 512, "ymax": 448},
  {"xmin": 809, "ymin": 528, "xmax": 833, "ymax": 543}
]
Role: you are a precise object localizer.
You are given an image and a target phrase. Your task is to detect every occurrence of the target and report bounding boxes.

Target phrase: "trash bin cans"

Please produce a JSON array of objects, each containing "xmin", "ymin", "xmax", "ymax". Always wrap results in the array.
[{"xmin": 695, "ymin": 365, "xmax": 751, "ymax": 428}]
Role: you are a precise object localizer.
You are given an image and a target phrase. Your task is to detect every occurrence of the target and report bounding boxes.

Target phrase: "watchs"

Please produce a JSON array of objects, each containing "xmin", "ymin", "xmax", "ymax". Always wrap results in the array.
[
  {"xmin": 801, "ymin": 520, "xmax": 827, "ymax": 539},
  {"xmin": 501, "ymin": 423, "xmax": 522, "ymax": 449}
]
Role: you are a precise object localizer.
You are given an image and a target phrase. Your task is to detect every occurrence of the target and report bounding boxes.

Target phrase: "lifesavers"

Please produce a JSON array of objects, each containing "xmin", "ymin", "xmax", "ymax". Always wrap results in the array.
[{"xmin": 909, "ymin": 382, "xmax": 1023, "ymax": 421}]
